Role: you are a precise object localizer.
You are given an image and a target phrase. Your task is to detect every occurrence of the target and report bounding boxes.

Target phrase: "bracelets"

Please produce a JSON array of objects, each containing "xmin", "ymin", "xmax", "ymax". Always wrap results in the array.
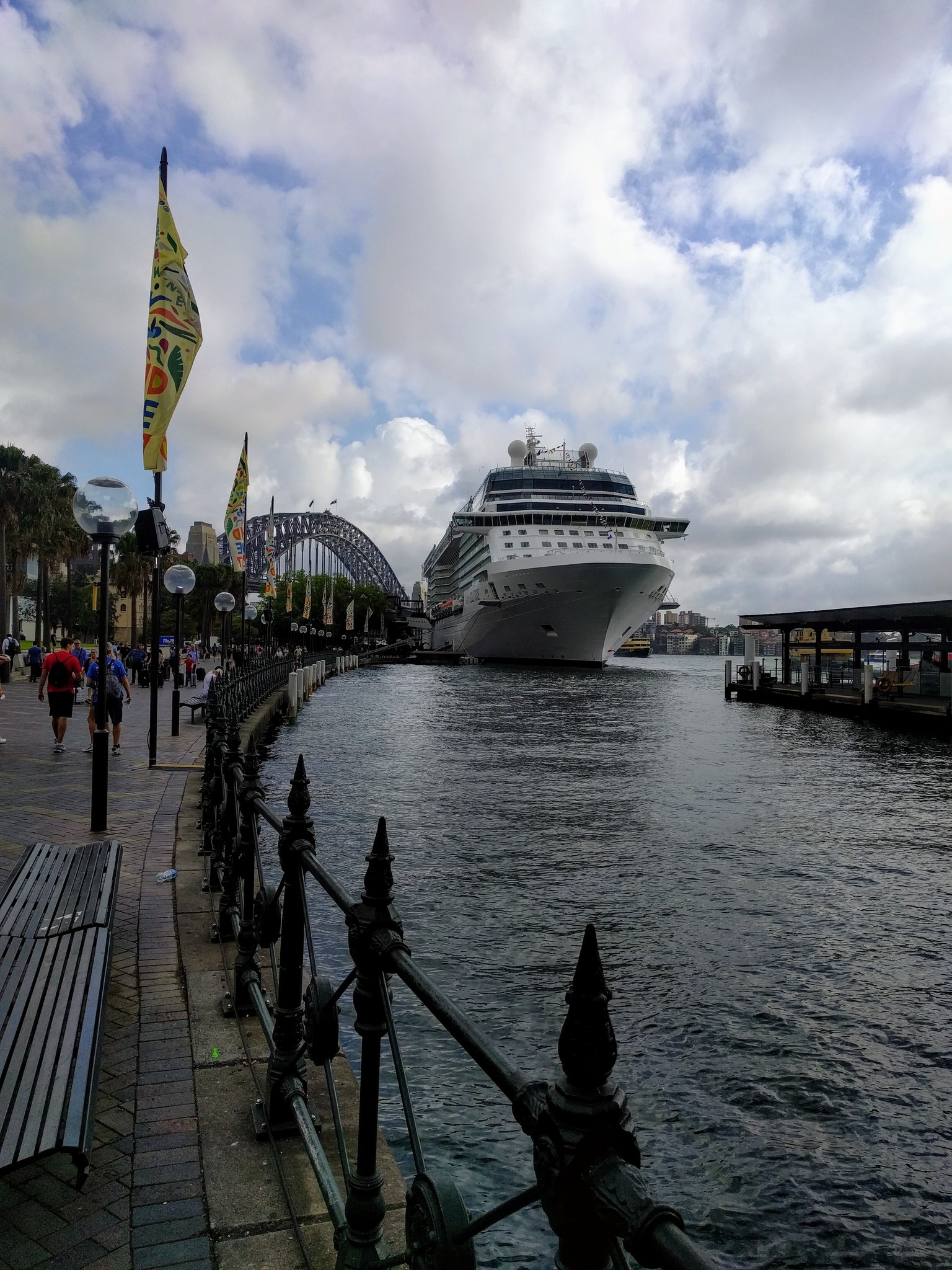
[{"xmin": 39, "ymin": 691, "xmax": 42, "ymax": 693}]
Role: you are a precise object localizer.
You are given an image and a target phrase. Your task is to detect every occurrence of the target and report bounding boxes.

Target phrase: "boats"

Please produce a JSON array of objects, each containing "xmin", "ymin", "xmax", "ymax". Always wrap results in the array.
[
  {"xmin": 788, "ymin": 628, "xmax": 901, "ymax": 672},
  {"xmin": 613, "ymin": 624, "xmax": 654, "ymax": 658},
  {"xmin": 431, "ymin": 599, "xmax": 452, "ymax": 618}
]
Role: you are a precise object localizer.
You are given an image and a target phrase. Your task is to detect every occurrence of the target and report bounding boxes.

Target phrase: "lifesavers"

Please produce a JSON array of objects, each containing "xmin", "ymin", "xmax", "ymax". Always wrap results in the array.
[
  {"xmin": 739, "ymin": 666, "xmax": 750, "ymax": 677},
  {"xmin": 876, "ymin": 677, "xmax": 892, "ymax": 692}
]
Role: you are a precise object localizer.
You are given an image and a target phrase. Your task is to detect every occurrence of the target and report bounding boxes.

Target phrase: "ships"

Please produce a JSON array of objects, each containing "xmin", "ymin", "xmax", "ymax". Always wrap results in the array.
[{"xmin": 420, "ymin": 422, "xmax": 692, "ymax": 669}]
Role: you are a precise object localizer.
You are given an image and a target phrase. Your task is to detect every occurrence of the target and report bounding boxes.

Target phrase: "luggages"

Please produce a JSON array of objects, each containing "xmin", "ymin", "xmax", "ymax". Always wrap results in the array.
[
  {"xmin": 174, "ymin": 664, "xmax": 184, "ymax": 687},
  {"xmin": 73, "ymin": 671, "xmax": 85, "ymax": 705},
  {"xmin": 137, "ymin": 660, "xmax": 150, "ymax": 688},
  {"xmin": 190, "ymin": 667, "xmax": 197, "ymax": 687},
  {"xmin": 158, "ymin": 669, "xmax": 164, "ymax": 688},
  {"xmin": 196, "ymin": 663, "xmax": 206, "ymax": 681},
  {"xmin": 123, "ymin": 660, "xmax": 128, "ymax": 678},
  {"xmin": 164, "ymin": 658, "xmax": 170, "ymax": 681}
]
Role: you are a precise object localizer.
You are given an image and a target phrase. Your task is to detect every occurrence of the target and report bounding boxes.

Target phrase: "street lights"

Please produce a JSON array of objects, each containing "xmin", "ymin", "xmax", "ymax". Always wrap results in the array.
[
  {"xmin": 310, "ymin": 628, "xmax": 316, "ymax": 656},
  {"xmin": 318, "ymin": 630, "xmax": 325, "ymax": 653},
  {"xmin": 261, "ymin": 613, "xmax": 274, "ymax": 658},
  {"xmin": 71, "ymin": 476, "xmax": 139, "ymax": 832},
  {"xmin": 326, "ymin": 632, "xmax": 332, "ymax": 648},
  {"xmin": 299, "ymin": 626, "xmax": 307, "ymax": 657},
  {"xmin": 244, "ymin": 605, "xmax": 257, "ymax": 667},
  {"xmin": 214, "ymin": 592, "xmax": 235, "ymax": 677},
  {"xmin": 341, "ymin": 635, "xmax": 346, "ymax": 655},
  {"xmin": 290, "ymin": 623, "xmax": 299, "ymax": 654},
  {"xmin": 163, "ymin": 564, "xmax": 196, "ymax": 736}
]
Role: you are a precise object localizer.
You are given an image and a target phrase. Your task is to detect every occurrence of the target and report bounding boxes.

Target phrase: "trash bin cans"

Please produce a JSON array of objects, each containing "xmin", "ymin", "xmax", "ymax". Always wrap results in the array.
[{"xmin": 0, "ymin": 654, "xmax": 11, "ymax": 684}]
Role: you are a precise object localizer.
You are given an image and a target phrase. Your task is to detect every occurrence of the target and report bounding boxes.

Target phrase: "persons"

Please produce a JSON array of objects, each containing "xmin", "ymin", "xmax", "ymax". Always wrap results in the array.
[
  {"xmin": 93, "ymin": 632, "xmax": 98, "ymax": 644},
  {"xmin": 2, "ymin": 634, "xmax": 20, "ymax": 674},
  {"xmin": 82, "ymin": 642, "xmax": 132, "ymax": 754},
  {"xmin": 98, "ymin": 640, "xmax": 343, "ymax": 697},
  {"xmin": 0, "ymin": 649, "xmax": 10, "ymax": 743},
  {"xmin": 20, "ymin": 630, "xmax": 26, "ymax": 640},
  {"xmin": 71, "ymin": 639, "xmax": 88, "ymax": 703},
  {"xmin": 38, "ymin": 637, "xmax": 82, "ymax": 752},
  {"xmin": 26, "ymin": 641, "xmax": 43, "ymax": 683},
  {"xmin": 51, "ymin": 631, "xmax": 57, "ymax": 653},
  {"xmin": 40, "ymin": 646, "xmax": 48, "ymax": 662},
  {"xmin": 81, "ymin": 649, "xmax": 99, "ymax": 704},
  {"xmin": 346, "ymin": 644, "xmax": 370, "ymax": 654}
]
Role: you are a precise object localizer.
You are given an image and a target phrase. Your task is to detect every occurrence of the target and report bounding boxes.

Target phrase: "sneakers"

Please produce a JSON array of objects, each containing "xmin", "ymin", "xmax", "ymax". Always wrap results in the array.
[
  {"xmin": 111, "ymin": 747, "xmax": 123, "ymax": 755},
  {"xmin": 53, "ymin": 744, "xmax": 67, "ymax": 752},
  {"xmin": 0, "ymin": 736, "xmax": 6, "ymax": 743},
  {"xmin": 82, "ymin": 743, "xmax": 93, "ymax": 752},
  {"xmin": 54, "ymin": 737, "xmax": 58, "ymax": 745},
  {"xmin": 85, "ymin": 699, "xmax": 91, "ymax": 704}
]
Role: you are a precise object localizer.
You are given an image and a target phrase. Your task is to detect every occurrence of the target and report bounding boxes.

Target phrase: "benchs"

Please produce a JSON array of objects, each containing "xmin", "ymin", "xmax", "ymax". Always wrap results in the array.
[
  {"xmin": 179, "ymin": 694, "xmax": 208, "ymax": 723},
  {"xmin": 828, "ymin": 671, "xmax": 883, "ymax": 686},
  {"xmin": 0, "ymin": 840, "xmax": 124, "ymax": 1192}
]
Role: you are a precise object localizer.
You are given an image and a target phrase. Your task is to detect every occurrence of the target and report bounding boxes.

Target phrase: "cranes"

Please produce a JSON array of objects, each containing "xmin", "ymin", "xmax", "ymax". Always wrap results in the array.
[{"xmin": 704, "ymin": 617, "xmax": 716, "ymax": 628}]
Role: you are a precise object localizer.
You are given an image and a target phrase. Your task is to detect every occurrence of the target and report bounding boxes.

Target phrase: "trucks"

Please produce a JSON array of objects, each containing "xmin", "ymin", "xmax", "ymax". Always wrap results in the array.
[
  {"xmin": 159, "ymin": 636, "xmax": 193, "ymax": 649},
  {"xmin": 194, "ymin": 636, "xmax": 221, "ymax": 649}
]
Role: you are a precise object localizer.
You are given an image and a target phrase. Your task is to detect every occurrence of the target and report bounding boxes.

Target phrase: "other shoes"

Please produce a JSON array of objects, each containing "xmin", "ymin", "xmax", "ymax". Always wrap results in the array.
[
  {"xmin": 32, "ymin": 681, "xmax": 38, "ymax": 683},
  {"xmin": 52, "ymin": 652, "xmax": 55, "ymax": 653},
  {"xmin": 29, "ymin": 680, "xmax": 32, "ymax": 683}
]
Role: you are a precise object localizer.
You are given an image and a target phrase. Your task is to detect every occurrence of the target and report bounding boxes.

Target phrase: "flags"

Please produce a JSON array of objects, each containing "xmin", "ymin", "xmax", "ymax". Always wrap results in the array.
[
  {"xmin": 381, "ymin": 611, "xmax": 384, "ymax": 634},
  {"xmin": 309, "ymin": 499, "xmax": 336, "ymax": 507},
  {"xmin": 286, "ymin": 542, "xmax": 292, "ymax": 612},
  {"xmin": 322, "ymin": 577, "xmax": 326, "ymax": 626},
  {"xmin": 266, "ymin": 504, "xmax": 277, "ymax": 599},
  {"xmin": 364, "ymin": 606, "xmax": 373, "ymax": 632},
  {"xmin": 141, "ymin": 169, "xmax": 202, "ymax": 473},
  {"xmin": 224, "ymin": 440, "xmax": 250, "ymax": 571},
  {"xmin": 346, "ymin": 599, "xmax": 354, "ymax": 630},
  {"xmin": 303, "ymin": 561, "xmax": 311, "ymax": 619},
  {"xmin": 325, "ymin": 586, "xmax": 334, "ymax": 624}
]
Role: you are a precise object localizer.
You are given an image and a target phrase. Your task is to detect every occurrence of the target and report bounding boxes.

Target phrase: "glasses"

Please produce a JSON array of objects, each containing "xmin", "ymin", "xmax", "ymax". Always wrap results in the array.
[
  {"xmin": 74, "ymin": 643, "xmax": 80, "ymax": 644},
  {"xmin": 191, "ymin": 646, "xmax": 194, "ymax": 648},
  {"xmin": 91, "ymin": 652, "xmax": 96, "ymax": 654}
]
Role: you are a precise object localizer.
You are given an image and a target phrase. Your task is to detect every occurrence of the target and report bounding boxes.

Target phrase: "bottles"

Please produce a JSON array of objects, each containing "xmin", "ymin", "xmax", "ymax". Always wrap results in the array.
[
  {"xmin": 156, "ymin": 869, "xmax": 178, "ymax": 882},
  {"xmin": 118, "ymin": 653, "xmax": 122, "ymax": 661},
  {"xmin": 117, "ymin": 650, "xmax": 120, "ymax": 654},
  {"xmin": 94, "ymin": 682, "xmax": 98, "ymax": 702}
]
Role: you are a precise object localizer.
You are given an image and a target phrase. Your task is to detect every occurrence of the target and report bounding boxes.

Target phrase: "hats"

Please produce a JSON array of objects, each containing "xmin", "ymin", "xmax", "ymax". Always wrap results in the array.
[{"xmin": 214, "ymin": 668, "xmax": 223, "ymax": 678}]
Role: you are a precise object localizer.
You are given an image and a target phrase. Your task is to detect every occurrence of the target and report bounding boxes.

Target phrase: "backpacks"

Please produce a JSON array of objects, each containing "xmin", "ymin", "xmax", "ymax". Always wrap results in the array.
[
  {"xmin": 6, "ymin": 638, "xmax": 18, "ymax": 654},
  {"xmin": 47, "ymin": 651, "xmax": 75, "ymax": 686},
  {"xmin": 95, "ymin": 659, "xmax": 121, "ymax": 701},
  {"xmin": 213, "ymin": 646, "xmax": 217, "ymax": 652}
]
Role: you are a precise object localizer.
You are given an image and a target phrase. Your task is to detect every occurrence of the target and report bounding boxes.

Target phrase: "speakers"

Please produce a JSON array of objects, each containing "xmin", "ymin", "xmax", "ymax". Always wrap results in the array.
[
  {"xmin": 133, "ymin": 509, "xmax": 169, "ymax": 553},
  {"xmin": 263, "ymin": 609, "xmax": 274, "ymax": 622}
]
